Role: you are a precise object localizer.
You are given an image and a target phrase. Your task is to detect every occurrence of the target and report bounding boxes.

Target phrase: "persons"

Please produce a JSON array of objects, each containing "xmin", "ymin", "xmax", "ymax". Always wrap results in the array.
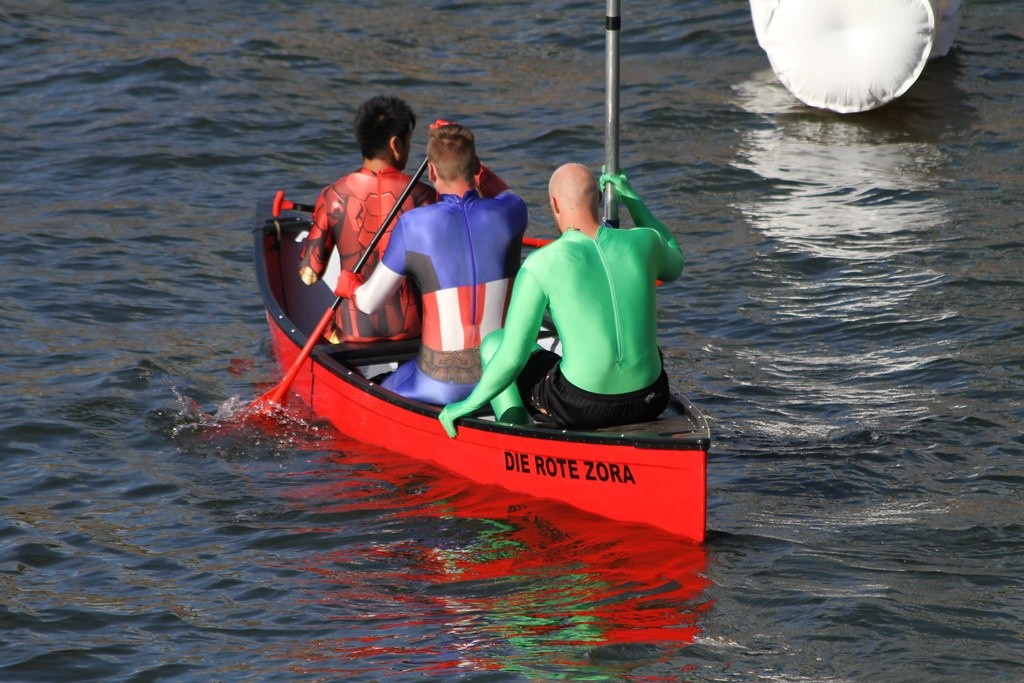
[
  {"xmin": 333, "ymin": 118, "xmax": 529, "ymax": 409},
  {"xmin": 297, "ymin": 95, "xmax": 446, "ymax": 345},
  {"xmin": 438, "ymin": 162, "xmax": 686, "ymax": 437}
]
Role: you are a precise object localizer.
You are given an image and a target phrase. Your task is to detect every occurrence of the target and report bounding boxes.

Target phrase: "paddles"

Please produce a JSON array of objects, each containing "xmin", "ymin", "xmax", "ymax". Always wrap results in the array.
[
  {"xmin": 268, "ymin": 190, "xmax": 663, "ymax": 288},
  {"xmin": 248, "ymin": 118, "xmax": 446, "ymax": 410}
]
